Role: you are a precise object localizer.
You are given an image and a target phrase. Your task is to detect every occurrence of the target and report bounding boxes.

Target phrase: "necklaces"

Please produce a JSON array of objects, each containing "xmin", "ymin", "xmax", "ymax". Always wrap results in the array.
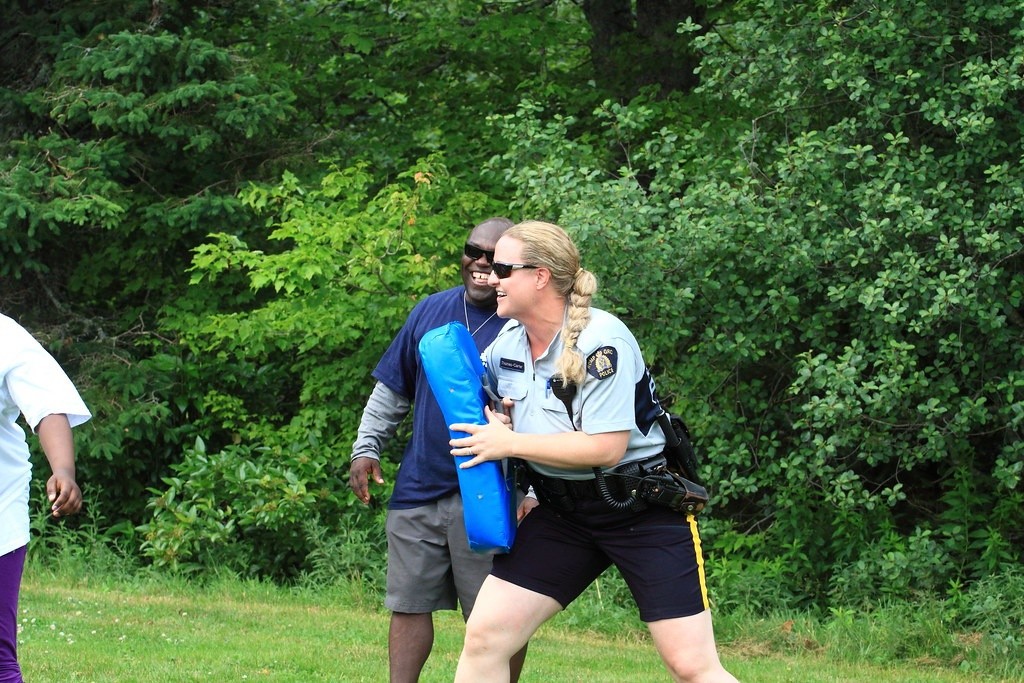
[{"xmin": 463, "ymin": 289, "xmax": 496, "ymax": 338}]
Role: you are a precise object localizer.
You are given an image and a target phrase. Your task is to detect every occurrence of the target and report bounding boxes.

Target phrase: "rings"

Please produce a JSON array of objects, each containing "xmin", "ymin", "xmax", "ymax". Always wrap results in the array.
[{"xmin": 469, "ymin": 448, "xmax": 473, "ymax": 456}]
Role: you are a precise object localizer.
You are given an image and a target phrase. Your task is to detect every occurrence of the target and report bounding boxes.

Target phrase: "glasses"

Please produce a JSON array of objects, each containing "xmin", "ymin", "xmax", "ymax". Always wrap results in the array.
[
  {"xmin": 488, "ymin": 259, "xmax": 537, "ymax": 279},
  {"xmin": 463, "ymin": 240, "xmax": 495, "ymax": 265}
]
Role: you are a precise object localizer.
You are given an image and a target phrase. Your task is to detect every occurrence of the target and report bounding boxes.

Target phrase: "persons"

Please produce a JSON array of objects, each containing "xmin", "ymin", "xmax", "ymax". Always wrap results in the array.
[
  {"xmin": 0, "ymin": 313, "xmax": 92, "ymax": 683},
  {"xmin": 448, "ymin": 219, "xmax": 743, "ymax": 682},
  {"xmin": 348, "ymin": 218, "xmax": 543, "ymax": 682}
]
